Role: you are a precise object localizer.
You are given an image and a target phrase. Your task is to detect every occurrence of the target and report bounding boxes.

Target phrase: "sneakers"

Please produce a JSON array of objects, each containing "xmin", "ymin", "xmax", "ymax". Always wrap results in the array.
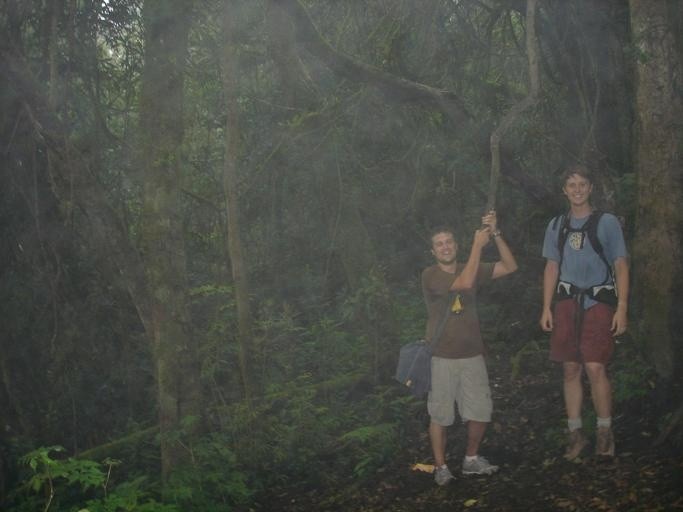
[
  {"xmin": 434, "ymin": 466, "xmax": 456, "ymax": 487},
  {"xmin": 563, "ymin": 425, "xmax": 616, "ymax": 466},
  {"xmin": 462, "ymin": 456, "xmax": 500, "ymax": 477}
]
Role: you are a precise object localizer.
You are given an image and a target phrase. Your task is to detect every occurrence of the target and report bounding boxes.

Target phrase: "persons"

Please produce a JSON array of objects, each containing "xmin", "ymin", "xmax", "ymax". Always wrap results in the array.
[
  {"xmin": 419, "ymin": 208, "xmax": 518, "ymax": 487},
  {"xmin": 538, "ymin": 164, "xmax": 630, "ymax": 462}
]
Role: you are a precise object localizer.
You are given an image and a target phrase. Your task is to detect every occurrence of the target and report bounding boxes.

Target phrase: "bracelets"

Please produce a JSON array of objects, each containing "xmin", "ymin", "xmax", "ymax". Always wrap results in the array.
[{"xmin": 489, "ymin": 229, "xmax": 504, "ymax": 237}]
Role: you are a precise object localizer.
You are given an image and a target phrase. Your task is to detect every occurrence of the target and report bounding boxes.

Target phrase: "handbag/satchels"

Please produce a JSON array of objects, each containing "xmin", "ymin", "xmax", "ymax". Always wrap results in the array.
[{"xmin": 395, "ymin": 339, "xmax": 432, "ymax": 400}]
